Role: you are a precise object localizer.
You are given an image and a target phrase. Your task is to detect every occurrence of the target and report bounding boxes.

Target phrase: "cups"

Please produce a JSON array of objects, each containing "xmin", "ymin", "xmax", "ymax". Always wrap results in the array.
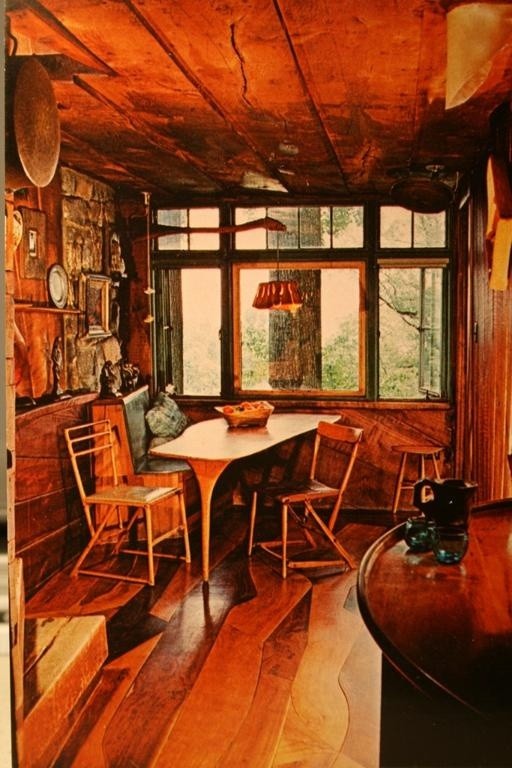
[
  {"xmin": 405, "ymin": 517, "xmax": 436, "ymax": 552},
  {"xmin": 435, "ymin": 530, "xmax": 469, "ymax": 566}
]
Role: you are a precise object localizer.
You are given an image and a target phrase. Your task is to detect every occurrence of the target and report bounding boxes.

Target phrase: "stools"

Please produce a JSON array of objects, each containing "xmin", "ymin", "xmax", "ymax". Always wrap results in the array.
[{"xmin": 390, "ymin": 445, "xmax": 447, "ymax": 515}]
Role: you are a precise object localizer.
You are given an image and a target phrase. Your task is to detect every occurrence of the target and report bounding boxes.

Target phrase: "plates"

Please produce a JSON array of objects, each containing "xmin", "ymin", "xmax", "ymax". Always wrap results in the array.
[{"xmin": 48, "ymin": 263, "xmax": 69, "ymax": 309}]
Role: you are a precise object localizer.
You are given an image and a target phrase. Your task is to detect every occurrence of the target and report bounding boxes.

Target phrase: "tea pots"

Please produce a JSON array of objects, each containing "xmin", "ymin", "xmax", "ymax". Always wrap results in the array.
[{"xmin": 413, "ymin": 478, "xmax": 478, "ymax": 531}]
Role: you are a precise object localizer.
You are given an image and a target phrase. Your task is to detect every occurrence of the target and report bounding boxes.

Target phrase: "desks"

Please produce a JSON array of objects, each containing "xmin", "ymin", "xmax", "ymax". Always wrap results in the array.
[
  {"xmin": 355, "ymin": 498, "xmax": 512, "ymax": 767},
  {"xmin": 148, "ymin": 412, "xmax": 342, "ymax": 582}
]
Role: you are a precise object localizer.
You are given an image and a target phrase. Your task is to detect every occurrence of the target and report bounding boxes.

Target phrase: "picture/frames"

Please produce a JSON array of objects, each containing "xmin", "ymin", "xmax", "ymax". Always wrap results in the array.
[
  {"xmin": 79, "ymin": 271, "xmax": 112, "ymax": 340},
  {"xmin": 18, "ymin": 204, "xmax": 48, "ymax": 280}
]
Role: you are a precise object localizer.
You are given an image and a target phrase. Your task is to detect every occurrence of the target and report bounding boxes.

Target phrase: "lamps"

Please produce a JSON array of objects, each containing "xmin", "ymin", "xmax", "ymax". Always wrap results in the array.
[{"xmin": 252, "ymin": 230, "xmax": 303, "ymax": 312}]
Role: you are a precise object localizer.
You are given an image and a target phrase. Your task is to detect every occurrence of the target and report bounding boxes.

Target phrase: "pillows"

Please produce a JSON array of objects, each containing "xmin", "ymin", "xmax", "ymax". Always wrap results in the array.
[
  {"xmin": 147, "ymin": 435, "xmax": 174, "ymax": 460},
  {"xmin": 144, "ymin": 391, "xmax": 187, "ymax": 438}
]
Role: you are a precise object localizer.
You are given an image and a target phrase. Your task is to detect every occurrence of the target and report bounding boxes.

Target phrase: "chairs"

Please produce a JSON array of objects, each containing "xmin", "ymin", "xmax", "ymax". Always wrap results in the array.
[
  {"xmin": 91, "ymin": 383, "xmax": 233, "ymax": 540},
  {"xmin": 64, "ymin": 418, "xmax": 192, "ymax": 587},
  {"xmin": 247, "ymin": 421, "xmax": 364, "ymax": 580}
]
